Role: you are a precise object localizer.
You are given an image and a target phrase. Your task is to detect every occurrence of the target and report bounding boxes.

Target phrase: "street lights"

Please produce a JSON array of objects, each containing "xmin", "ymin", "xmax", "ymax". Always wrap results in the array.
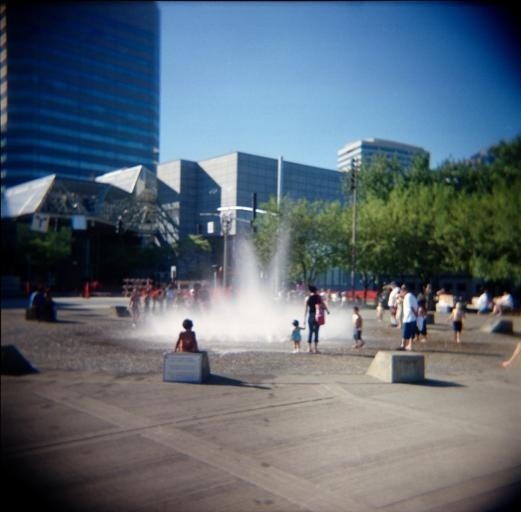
[
  {"xmin": 351, "ymin": 158, "xmax": 358, "ymax": 298},
  {"xmin": 220, "ymin": 212, "xmax": 231, "ymax": 288}
]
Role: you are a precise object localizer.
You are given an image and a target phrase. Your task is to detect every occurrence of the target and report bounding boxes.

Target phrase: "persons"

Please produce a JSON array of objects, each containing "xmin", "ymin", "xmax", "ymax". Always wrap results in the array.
[
  {"xmin": 289, "ymin": 285, "xmax": 365, "ymax": 352},
  {"xmin": 128, "ymin": 282, "xmax": 211, "ymax": 329},
  {"xmin": 175, "ymin": 319, "xmax": 198, "ymax": 352},
  {"xmin": 27, "ymin": 284, "xmax": 50, "ymax": 322},
  {"xmin": 377, "ymin": 279, "xmax": 513, "ymax": 350},
  {"xmin": 44, "ymin": 288, "xmax": 59, "ymax": 321},
  {"xmin": 502, "ymin": 342, "xmax": 521, "ymax": 369}
]
